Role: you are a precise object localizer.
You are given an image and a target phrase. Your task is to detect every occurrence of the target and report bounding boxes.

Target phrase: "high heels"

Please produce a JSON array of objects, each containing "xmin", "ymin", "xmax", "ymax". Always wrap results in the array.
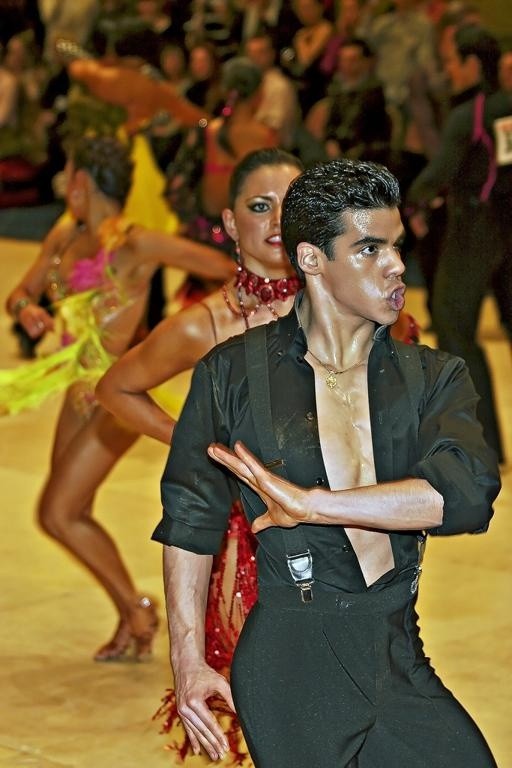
[{"xmin": 94, "ymin": 592, "xmax": 161, "ymax": 661}]
